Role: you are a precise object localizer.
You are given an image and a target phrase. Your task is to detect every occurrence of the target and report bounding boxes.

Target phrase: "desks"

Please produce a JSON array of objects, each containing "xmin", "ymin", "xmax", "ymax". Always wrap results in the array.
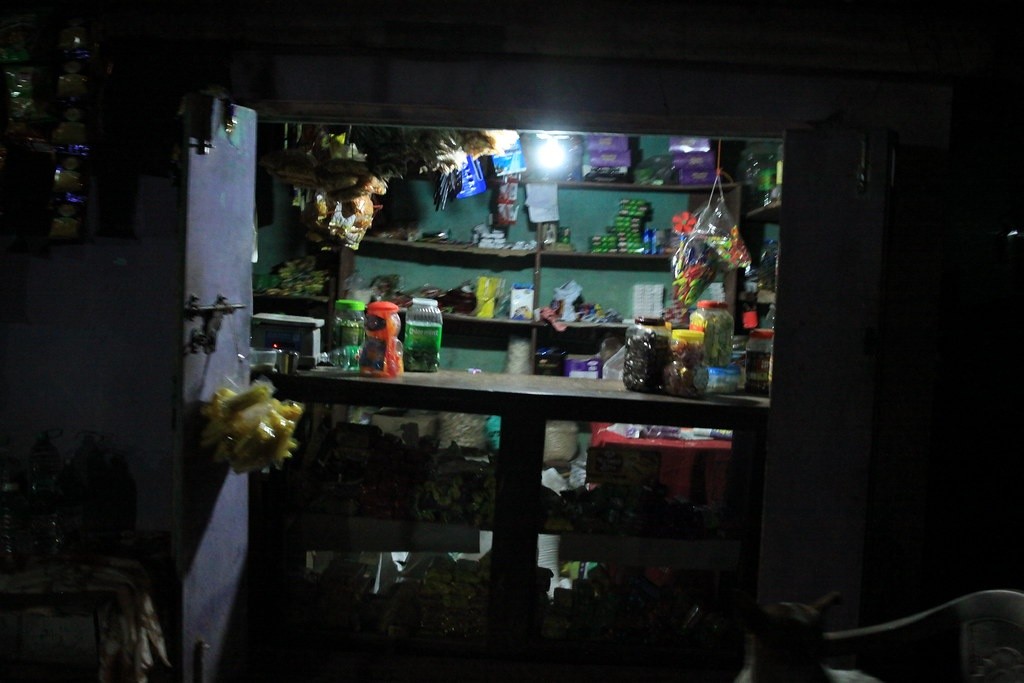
[{"xmin": 278, "ymin": 371, "xmax": 772, "ymax": 662}]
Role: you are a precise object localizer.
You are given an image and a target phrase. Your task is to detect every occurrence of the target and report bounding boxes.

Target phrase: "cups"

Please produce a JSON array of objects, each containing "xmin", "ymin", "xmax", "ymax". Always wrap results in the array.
[{"xmin": 279, "ymin": 350, "xmax": 300, "ymax": 374}]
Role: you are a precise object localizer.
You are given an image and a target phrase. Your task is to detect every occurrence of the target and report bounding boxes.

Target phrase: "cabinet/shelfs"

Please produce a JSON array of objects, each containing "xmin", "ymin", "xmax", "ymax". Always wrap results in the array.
[{"xmin": 247, "ymin": 103, "xmax": 788, "ymax": 662}]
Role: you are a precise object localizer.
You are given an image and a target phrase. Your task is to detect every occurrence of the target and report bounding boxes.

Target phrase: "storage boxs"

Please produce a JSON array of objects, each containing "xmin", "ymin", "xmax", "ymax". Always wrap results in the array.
[
  {"xmin": 588, "ymin": 133, "xmax": 632, "ymax": 182},
  {"xmin": 668, "ymin": 133, "xmax": 718, "ymax": 186}
]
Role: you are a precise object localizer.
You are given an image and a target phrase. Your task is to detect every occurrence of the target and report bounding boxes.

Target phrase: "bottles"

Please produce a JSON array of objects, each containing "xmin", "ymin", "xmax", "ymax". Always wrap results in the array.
[
  {"xmin": 362, "ymin": 300, "xmax": 404, "ymax": 377},
  {"xmin": 403, "ymin": 297, "xmax": 442, "ymax": 373},
  {"xmin": 0, "ymin": 433, "xmax": 32, "ymax": 571},
  {"xmin": 331, "ymin": 298, "xmax": 366, "ymax": 373},
  {"xmin": 33, "ymin": 431, "xmax": 64, "ymax": 549},
  {"xmin": 759, "ymin": 240, "xmax": 776, "ymax": 266},
  {"xmin": 0, "ymin": 484, "xmax": 23, "ymax": 576}
]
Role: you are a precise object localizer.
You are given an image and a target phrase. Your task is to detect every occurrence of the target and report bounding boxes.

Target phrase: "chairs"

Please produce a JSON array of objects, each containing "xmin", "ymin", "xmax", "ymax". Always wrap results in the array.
[{"xmin": 732, "ymin": 588, "xmax": 1024, "ymax": 683}]
